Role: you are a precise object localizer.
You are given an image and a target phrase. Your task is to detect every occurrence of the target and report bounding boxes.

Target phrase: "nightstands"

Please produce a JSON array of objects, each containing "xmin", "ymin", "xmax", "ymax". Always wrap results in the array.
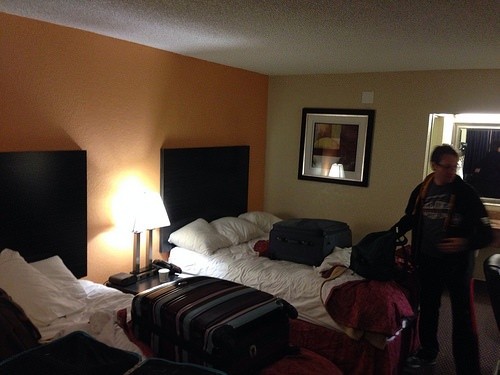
[{"xmin": 104, "ymin": 272, "xmax": 178, "ymax": 294}]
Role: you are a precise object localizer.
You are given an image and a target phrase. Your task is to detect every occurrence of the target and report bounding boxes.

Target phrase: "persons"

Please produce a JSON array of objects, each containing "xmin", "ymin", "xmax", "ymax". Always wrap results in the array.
[
  {"xmin": 389, "ymin": 144, "xmax": 496, "ymax": 375},
  {"xmin": 472, "ymin": 140, "xmax": 500, "ymax": 199}
]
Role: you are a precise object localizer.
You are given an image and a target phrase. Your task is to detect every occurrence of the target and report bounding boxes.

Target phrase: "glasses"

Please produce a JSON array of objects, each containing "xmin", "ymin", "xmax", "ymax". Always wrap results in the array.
[{"xmin": 437, "ymin": 162, "xmax": 459, "ymax": 169}]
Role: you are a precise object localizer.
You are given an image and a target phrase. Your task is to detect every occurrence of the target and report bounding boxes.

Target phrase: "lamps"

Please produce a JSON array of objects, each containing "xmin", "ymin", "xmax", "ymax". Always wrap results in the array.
[{"xmin": 107, "ymin": 192, "xmax": 171, "ymax": 284}]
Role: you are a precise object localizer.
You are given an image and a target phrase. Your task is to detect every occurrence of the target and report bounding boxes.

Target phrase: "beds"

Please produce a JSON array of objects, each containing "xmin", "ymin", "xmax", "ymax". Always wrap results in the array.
[
  {"xmin": 161, "ymin": 145, "xmax": 420, "ymax": 375},
  {"xmin": 0, "ymin": 150, "xmax": 346, "ymax": 375}
]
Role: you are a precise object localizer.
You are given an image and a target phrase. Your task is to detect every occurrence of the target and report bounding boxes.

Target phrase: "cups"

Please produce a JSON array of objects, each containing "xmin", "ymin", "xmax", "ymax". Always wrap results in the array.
[{"xmin": 158, "ymin": 268, "xmax": 170, "ymax": 283}]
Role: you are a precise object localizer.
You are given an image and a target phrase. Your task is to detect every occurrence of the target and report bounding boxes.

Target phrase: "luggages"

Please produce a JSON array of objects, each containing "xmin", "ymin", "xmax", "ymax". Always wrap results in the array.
[
  {"xmin": 132, "ymin": 275, "xmax": 300, "ymax": 375},
  {"xmin": 268, "ymin": 218, "xmax": 352, "ymax": 267}
]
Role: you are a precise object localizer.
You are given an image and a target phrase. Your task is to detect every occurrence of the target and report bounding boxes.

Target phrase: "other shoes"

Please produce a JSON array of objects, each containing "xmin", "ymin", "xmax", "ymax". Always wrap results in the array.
[{"xmin": 405, "ymin": 346, "xmax": 441, "ymax": 368}]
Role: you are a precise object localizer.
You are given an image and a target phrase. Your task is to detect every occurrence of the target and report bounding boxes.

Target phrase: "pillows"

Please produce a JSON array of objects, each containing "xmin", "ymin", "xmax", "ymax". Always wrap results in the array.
[
  {"xmin": 167, "ymin": 218, "xmax": 231, "ymax": 257},
  {"xmin": 0, "ymin": 248, "xmax": 85, "ymax": 327},
  {"xmin": 210, "ymin": 217, "xmax": 265, "ymax": 245},
  {"xmin": 29, "ymin": 255, "xmax": 87, "ymax": 299},
  {"xmin": 238, "ymin": 211, "xmax": 283, "ymax": 232}
]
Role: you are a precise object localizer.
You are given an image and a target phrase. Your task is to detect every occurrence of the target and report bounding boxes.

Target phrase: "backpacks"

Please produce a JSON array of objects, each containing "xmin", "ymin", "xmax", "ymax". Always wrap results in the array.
[{"xmin": 348, "ymin": 231, "xmax": 408, "ymax": 282}]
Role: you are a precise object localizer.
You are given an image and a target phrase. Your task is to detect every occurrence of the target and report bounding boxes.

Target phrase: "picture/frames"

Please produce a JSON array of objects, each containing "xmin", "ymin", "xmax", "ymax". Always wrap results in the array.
[{"xmin": 297, "ymin": 108, "xmax": 377, "ymax": 187}]
